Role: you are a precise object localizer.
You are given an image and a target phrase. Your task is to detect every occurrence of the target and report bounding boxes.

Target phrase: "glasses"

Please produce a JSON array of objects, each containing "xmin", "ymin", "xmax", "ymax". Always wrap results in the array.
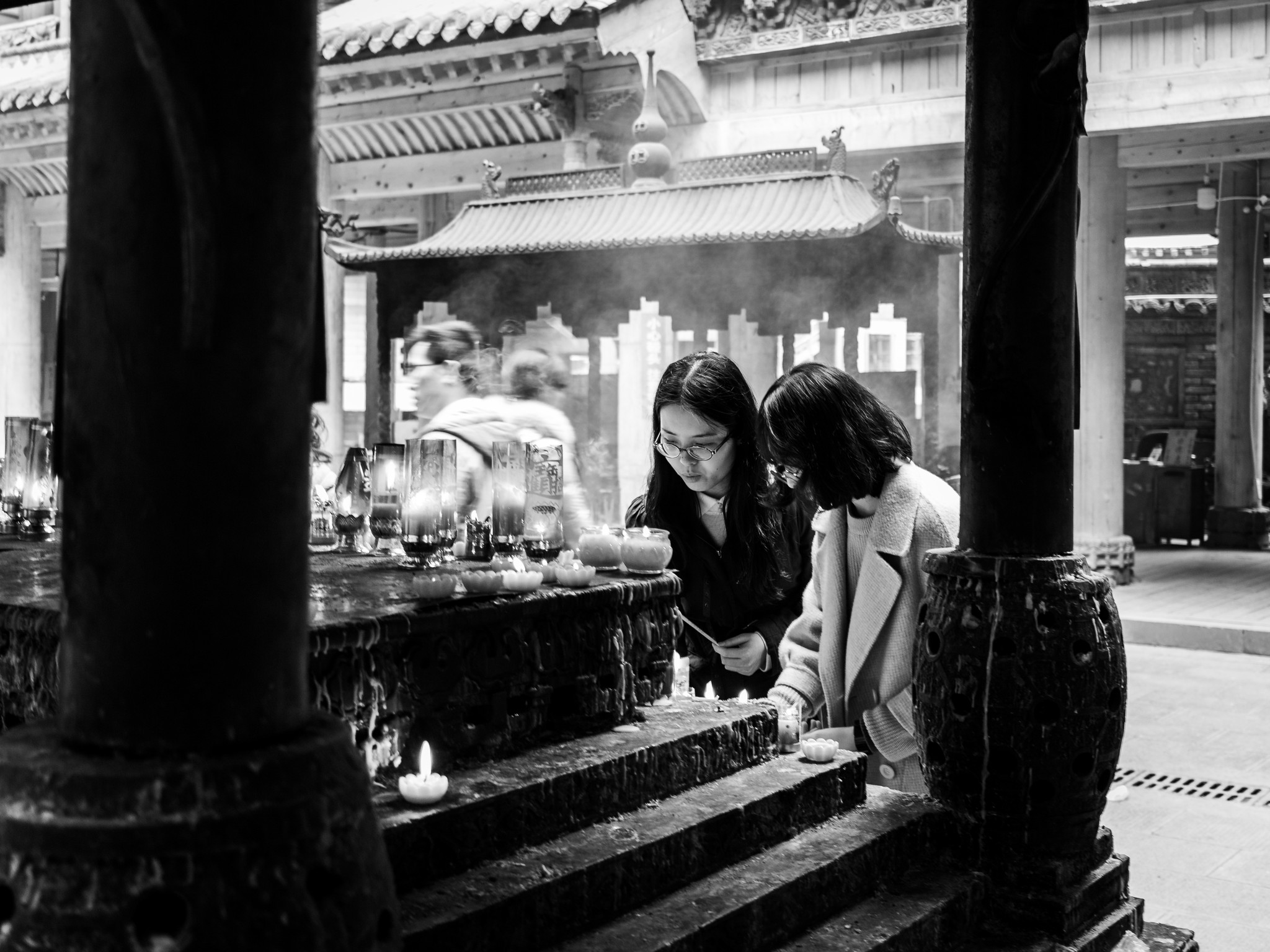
[
  {"xmin": 770, "ymin": 467, "xmax": 801, "ymax": 485},
  {"xmin": 653, "ymin": 430, "xmax": 730, "ymax": 461}
]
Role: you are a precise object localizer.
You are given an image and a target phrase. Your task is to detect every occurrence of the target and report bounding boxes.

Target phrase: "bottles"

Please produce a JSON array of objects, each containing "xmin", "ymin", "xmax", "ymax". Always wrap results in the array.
[{"xmin": 577, "ymin": 526, "xmax": 623, "ymax": 570}]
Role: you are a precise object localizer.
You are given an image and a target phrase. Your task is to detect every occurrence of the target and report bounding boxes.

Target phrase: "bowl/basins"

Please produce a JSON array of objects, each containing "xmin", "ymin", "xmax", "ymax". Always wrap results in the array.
[
  {"xmin": 412, "ymin": 574, "xmax": 456, "ymax": 600},
  {"xmin": 462, "ymin": 556, "xmax": 598, "ymax": 593},
  {"xmin": 800, "ymin": 738, "xmax": 838, "ymax": 762},
  {"xmin": 398, "ymin": 772, "xmax": 449, "ymax": 804}
]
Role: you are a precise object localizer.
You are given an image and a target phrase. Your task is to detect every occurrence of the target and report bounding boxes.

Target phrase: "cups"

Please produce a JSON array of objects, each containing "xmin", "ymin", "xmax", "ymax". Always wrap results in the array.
[
  {"xmin": 398, "ymin": 439, "xmax": 444, "ymax": 570},
  {"xmin": 777, "ymin": 703, "xmax": 802, "ymax": 752},
  {"xmin": 421, "ymin": 439, "xmax": 457, "ymax": 562},
  {"xmin": 368, "ymin": 442, "xmax": 404, "ymax": 556},
  {"xmin": 522, "ymin": 442, "xmax": 568, "ymax": 561},
  {"xmin": 492, "ymin": 440, "xmax": 526, "ymax": 557},
  {"xmin": 0, "ymin": 416, "xmax": 57, "ymax": 542},
  {"xmin": 620, "ymin": 527, "xmax": 673, "ymax": 575},
  {"xmin": 333, "ymin": 448, "xmax": 371, "ymax": 553}
]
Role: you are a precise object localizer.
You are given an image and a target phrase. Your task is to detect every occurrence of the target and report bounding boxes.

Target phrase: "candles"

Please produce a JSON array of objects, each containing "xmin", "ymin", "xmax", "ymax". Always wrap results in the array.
[
  {"xmin": 399, "ymin": 741, "xmax": 448, "ymax": 804},
  {"xmin": 0, "ymin": 415, "xmax": 674, "ymax": 598},
  {"xmin": 674, "ymin": 650, "xmax": 839, "ymax": 761}
]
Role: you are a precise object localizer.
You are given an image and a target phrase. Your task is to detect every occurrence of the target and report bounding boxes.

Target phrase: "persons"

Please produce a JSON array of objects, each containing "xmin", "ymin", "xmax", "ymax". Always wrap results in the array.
[
  {"xmin": 755, "ymin": 361, "xmax": 960, "ymax": 802},
  {"xmin": 622, "ymin": 348, "xmax": 824, "ymax": 700},
  {"xmin": 395, "ymin": 319, "xmax": 600, "ymax": 555}
]
